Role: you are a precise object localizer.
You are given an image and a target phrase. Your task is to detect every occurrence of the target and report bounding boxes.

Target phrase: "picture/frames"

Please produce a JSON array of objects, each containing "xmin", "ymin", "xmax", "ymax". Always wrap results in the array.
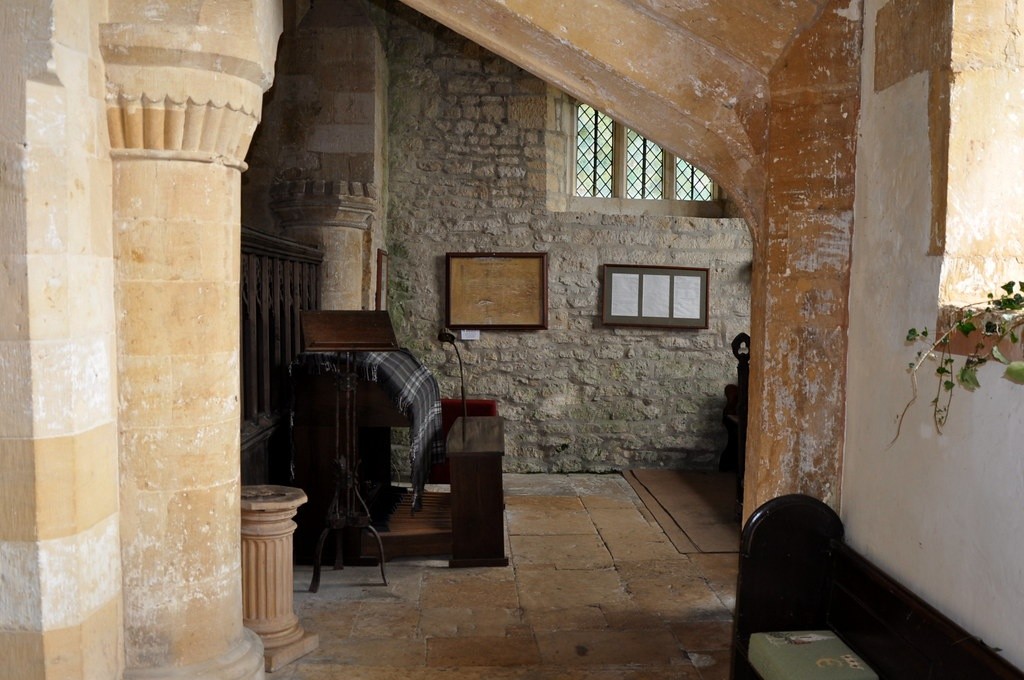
[
  {"xmin": 602, "ymin": 264, "xmax": 710, "ymax": 329},
  {"xmin": 446, "ymin": 252, "xmax": 548, "ymax": 330},
  {"xmin": 376, "ymin": 248, "xmax": 389, "ymax": 311}
]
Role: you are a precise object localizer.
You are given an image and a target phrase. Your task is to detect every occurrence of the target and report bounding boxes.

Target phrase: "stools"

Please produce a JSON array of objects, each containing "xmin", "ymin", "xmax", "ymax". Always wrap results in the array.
[{"xmin": 445, "ymin": 416, "xmax": 509, "ymax": 568}]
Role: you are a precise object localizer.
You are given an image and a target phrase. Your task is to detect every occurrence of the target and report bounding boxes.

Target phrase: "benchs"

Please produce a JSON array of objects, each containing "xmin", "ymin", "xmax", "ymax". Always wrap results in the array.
[{"xmin": 729, "ymin": 494, "xmax": 1024, "ymax": 680}]
